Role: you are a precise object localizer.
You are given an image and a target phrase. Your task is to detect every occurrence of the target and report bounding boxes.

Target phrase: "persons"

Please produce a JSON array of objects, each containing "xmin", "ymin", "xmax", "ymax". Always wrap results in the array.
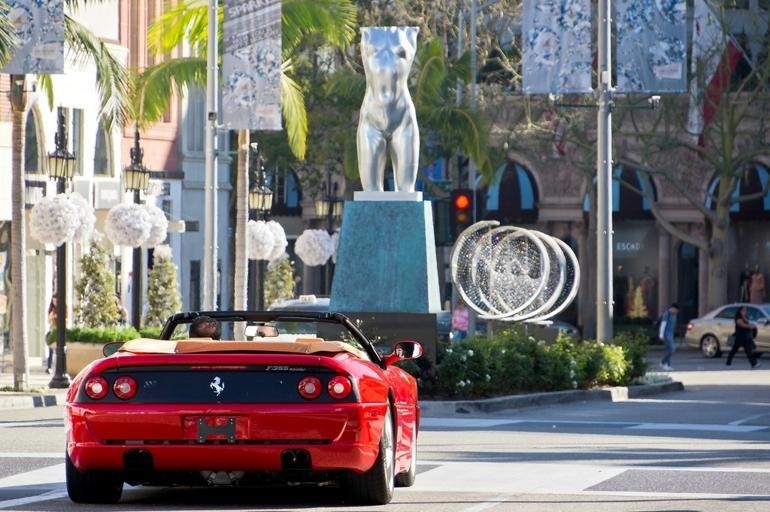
[
  {"xmin": 45, "ymin": 294, "xmax": 58, "ymax": 374},
  {"xmin": 726, "ymin": 306, "xmax": 760, "ymax": 368},
  {"xmin": 658, "ymin": 303, "xmax": 680, "ymax": 371},
  {"xmin": 749, "ymin": 264, "xmax": 766, "ymax": 304},
  {"xmin": 613, "ymin": 265, "xmax": 628, "ymax": 315},
  {"xmin": 357, "ymin": 26, "xmax": 420, "ymax": 193},
  {"xmin": 639, "ymin": 266, "xmax": 656, "ymax": 306},
  {"xmin": 451, "ymin": 299, "xmax": 469, "ymax": 341},
  {"xmin": 739, "ymin": 263, "xmax": 752, "ymax": 302},
  {"xmin": 189, "ymin": 316, "xmax": 222, "ymax": 340}
]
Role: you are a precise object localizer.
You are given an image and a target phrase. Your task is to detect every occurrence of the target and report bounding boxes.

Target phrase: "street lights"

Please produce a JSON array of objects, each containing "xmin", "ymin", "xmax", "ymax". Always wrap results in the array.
[
  {"xmin": 245, "ymin": 157, "xmax": 288, "ymax": 310},
  {"xmin": 28, "ymin": 107, "xmax": 96, "ymax": 376},
  {"xmin": 105, "ymin": 130, "xmax": 168, "ymax": 332},
  {"xmin": 548, "ymin": 93, "xmax": 661, "ymax": 341},
  {"xmin": 294, "ymin": 170, "xmax": 344, "ymax": 295}
]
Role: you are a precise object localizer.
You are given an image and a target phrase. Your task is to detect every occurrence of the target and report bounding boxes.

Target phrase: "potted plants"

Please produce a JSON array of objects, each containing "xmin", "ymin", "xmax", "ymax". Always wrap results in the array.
[{"xmin": 45, "ymin": 325, "xmax": 139, "ymax": 380}]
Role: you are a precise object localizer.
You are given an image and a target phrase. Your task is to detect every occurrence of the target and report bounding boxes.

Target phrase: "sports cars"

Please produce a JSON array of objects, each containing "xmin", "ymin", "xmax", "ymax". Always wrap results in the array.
[{"xmin": 64, "ymin": 310, "xmax": 424, "ymax": 502}]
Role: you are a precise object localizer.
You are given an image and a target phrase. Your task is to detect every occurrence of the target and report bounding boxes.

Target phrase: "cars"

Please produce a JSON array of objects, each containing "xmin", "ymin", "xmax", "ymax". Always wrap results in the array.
[{"xmin": 684, "ymin": 303, "xmax": 770, "ymax": 358}]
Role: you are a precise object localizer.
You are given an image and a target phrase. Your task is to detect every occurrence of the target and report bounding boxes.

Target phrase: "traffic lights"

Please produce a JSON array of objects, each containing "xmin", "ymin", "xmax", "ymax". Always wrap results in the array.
[{"xmin": 448, "ymin": 188, "xmax": 476, "ymax": 245}]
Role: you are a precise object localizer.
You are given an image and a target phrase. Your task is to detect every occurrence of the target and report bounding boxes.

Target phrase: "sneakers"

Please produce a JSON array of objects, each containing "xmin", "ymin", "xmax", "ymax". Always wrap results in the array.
[
  {"xmin": 659, "ymin": 362, "xmax": 672, "ymax": 371},
  {"xmin": 752, "ymin": 363, "xmax": 760, "ymax": 368}
]
